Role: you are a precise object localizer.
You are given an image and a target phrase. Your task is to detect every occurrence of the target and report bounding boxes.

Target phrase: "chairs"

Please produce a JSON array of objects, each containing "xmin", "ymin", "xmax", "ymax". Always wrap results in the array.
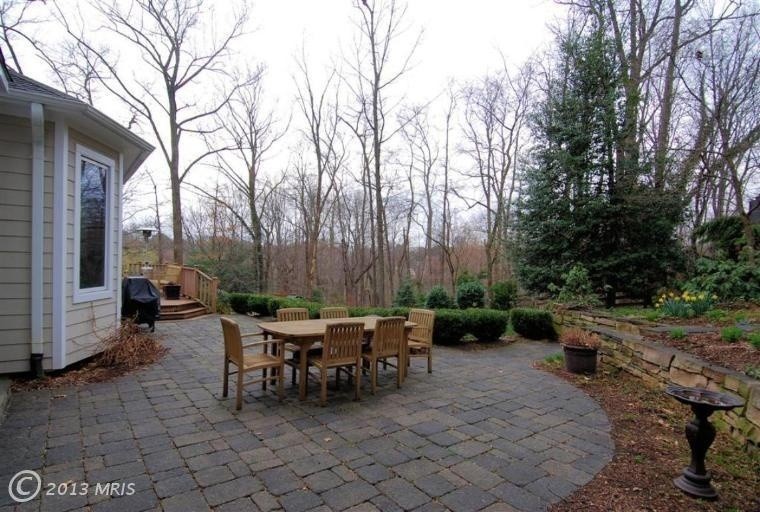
[
  {"xmin": 219, "ymin": 307, "xmax": 436, "ymax": 411},
  {"xmin": 150, "ymin": 264, "xmax": 182, "ymax": 297}
]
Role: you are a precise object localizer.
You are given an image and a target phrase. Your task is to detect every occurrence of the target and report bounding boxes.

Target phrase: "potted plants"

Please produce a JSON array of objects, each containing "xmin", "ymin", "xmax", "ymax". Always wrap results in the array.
[{"xmin": 561, "ymin": 327, "xmax": 601, "ymax": 374}]
